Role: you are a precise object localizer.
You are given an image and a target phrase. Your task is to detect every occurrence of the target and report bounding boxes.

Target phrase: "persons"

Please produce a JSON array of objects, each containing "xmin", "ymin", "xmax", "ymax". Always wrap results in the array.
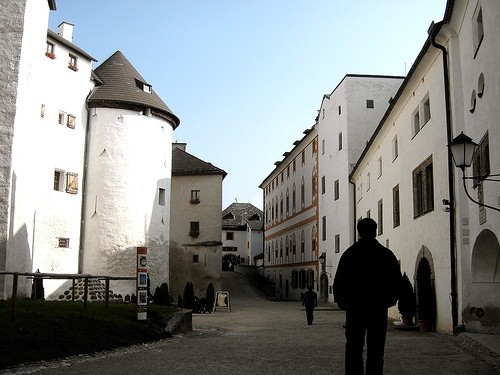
[
  {"xmin": 302, "ymin": 284, "xmax": 318, "ymax": 325},
  {"xmin": 332, "ymin": 218, "xmax": 404, "ymax": 375}
]
